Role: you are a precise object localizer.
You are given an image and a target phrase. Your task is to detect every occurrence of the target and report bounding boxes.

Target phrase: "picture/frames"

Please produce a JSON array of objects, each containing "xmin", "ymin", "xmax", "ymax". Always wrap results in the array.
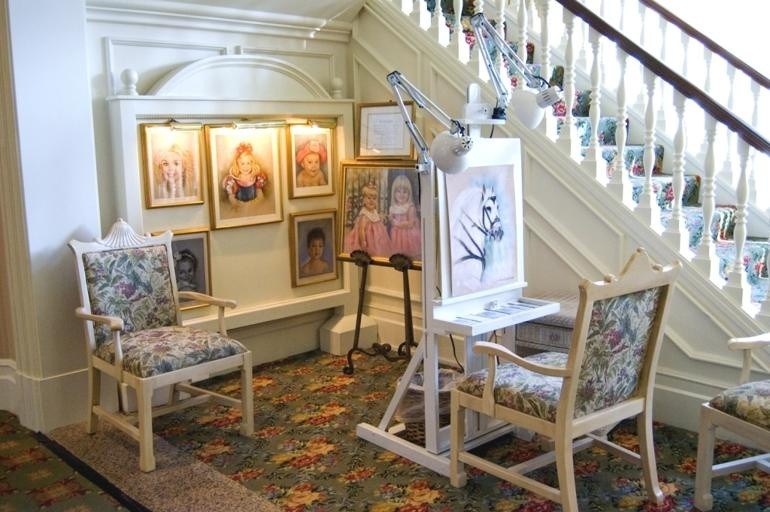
[
  {"xmin": 139, "ymin": 118, "xmax": 204, "ymax": 210},
  {"xmin": 354, "ymin": 98, "xmax": 417, "ymax": 161},
  {"xmin": 144, "ymin": 227, "xmax": 213, "ymax": 311},
  {"xmin": 288, "ymin": 208, "xmax": 339, "ymax": 288},
  {"xmin": 337, "ymin": 160, "xmax": 423, "ymax": 270},
  {"xmin": 204, "ymin": 119, "xmax": 284, "ymax": 229},
  {"xmin": 286, "ymin": 122, "xmax": 337, "ymax": 199}
]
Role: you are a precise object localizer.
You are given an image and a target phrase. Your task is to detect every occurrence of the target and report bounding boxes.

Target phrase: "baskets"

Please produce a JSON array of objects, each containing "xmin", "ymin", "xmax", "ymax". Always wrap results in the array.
[{"xmin": 394, "ymin": 402, "xmax": 451, "ymax": 444}]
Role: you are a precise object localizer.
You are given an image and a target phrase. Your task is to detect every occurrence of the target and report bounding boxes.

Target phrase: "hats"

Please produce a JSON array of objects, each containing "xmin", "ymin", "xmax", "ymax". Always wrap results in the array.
[{"xmin": 296, "ymin": 140, "xmax": 326, "ymax": 166}]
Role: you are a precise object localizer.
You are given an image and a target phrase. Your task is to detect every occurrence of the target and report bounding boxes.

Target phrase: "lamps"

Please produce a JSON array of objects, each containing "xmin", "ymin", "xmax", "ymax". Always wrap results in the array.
[
  {"xmin": 387, "ymin": 66, "xmax": 474, "ymax": 176},
  {"xmin": 469, "ymin": 11, "xmax": 563, "ymax": 130}
]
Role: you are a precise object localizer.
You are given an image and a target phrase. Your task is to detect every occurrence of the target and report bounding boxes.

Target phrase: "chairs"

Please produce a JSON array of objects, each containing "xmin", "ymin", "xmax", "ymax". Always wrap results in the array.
[
  {"xmin": 449, "ymin": 249, "xmax": 682, "ymax": 512},
  {"xmin": 693, "ymin": 331, "xmax": 770, "ymax": 511},
  {"xmin": 68, "ymin": 218, "xmax": 255, "ymax": 472}
]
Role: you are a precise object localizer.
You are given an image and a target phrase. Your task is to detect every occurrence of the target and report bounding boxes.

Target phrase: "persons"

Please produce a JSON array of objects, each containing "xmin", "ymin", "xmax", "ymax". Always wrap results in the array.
[
  {"xmin": 155, "ymin": 142, "xmax": 195, "ymax": 197},
  {"xmin": 387, "ymin": 175, "xmax": 421, "ymax": 259},
  {"xmin": 301, "ymin": 227, "xmax": 329, "ymax": 273},
  {"xmin": 173, "ymin": 249, "xmax": 199, "ymax": 302},
  {"xmin": 295, "ymin": 139, "xmax": 328, "ymax": 187},
  {"xmin": 222, "ymin": 142, "xmax": 268, "ymax": 208},
  {"xmin": 345, "ymin": 186, "xmax": 391, "ymax": 257}
]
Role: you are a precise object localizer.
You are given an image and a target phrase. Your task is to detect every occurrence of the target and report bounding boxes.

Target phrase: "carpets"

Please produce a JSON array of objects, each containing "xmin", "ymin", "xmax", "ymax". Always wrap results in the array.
[
  {"xmin": 135, "ymin": 345, "xmax": 770, "ymax": 510},
  {"xmin": 1, "ymin": 407, "xmax": 151, "ymax": 511}
]
